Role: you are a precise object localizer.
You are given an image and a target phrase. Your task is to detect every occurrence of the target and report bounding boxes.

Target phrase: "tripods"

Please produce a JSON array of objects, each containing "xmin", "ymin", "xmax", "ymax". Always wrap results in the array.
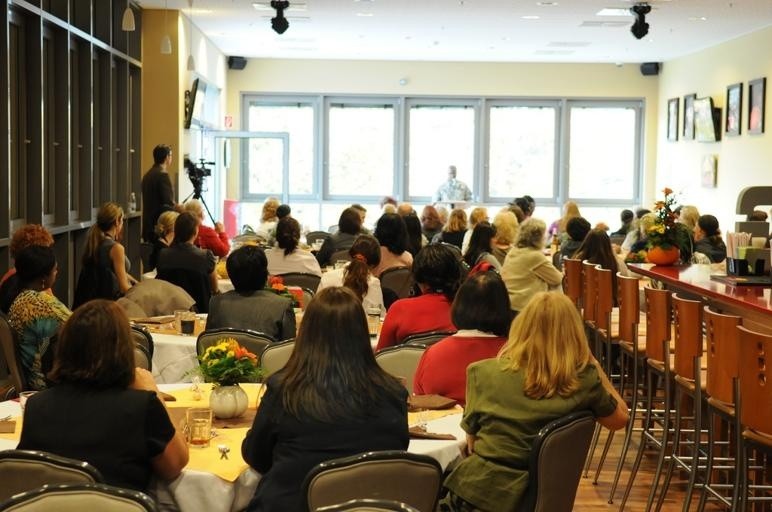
[{"xmin": 182, "ymin": 191, "xmax": 216, "ymax": 226}]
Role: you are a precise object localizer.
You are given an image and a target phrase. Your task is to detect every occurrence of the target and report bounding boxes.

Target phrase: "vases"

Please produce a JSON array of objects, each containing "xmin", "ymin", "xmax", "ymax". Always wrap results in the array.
[
  {"xmin": 647, "ymin": 243, "xmax": 682, "ymax": 266},
  {"xmin": 209, "ymin": 384, "xmax": 248, "ymax": 419}
]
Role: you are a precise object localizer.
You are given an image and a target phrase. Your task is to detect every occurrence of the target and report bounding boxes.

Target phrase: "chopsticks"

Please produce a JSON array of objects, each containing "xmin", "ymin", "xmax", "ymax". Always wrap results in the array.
[{"xmin": 727, "ymin": 231, "xmax": 751, "ymax": 259}]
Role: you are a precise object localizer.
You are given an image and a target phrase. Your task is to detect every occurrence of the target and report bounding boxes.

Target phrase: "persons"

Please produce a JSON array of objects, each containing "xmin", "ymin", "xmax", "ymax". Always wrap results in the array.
[
  {"xmin": 432, "ymin": 165, "xmax": 473, "ymax": 202},
  {"xmin": 141, "ymin": 143, "xmax": 175, "ymax": 243}
]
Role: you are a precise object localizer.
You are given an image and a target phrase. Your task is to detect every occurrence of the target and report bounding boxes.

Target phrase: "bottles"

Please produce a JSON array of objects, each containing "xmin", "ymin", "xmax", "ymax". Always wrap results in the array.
[
  {"xmin": 131, "ymin": 192, "xmax": 137, "ymax": 212},
  {"xmin": 191, "ymin": 376, "xmax": 203, "ymax": 401},
  {"xmin": 550, "ymin": 228, "xmax": 560, "ymax": 258},
  {"xmin": 367, "ymin": 308, "xmax": 381, "ymax": 337}
]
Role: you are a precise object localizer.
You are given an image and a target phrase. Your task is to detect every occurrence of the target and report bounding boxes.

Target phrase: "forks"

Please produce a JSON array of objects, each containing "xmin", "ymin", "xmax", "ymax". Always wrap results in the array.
[{"xmin": 219, "ymin": 447, "xmax": 230, "ymax": 460}]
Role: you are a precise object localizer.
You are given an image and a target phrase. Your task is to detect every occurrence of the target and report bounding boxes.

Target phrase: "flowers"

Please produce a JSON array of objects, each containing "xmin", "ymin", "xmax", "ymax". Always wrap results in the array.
[
  {"xmin": 202, "ymin": 336, "xmax": 258, "ymax": 366},
  {"xmin": 640, "ymin": 186, "xmax": 699, "ymax": 252}
]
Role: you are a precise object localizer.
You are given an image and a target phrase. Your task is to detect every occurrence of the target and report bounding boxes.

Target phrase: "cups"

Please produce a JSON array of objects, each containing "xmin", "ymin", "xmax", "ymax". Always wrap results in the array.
[
  {"xmin": 184, "ymin": 408, "xmax": 213, "ymax": 449},
  {"xmin": 174, "ymin": 309, "xmax": 196, "ymax": 335},
  {"xmin": 312, "ymin": 239, "xmax": 324, "ymax": 255},
  {"xmin": 225, "ymin": 115, "xmax": 232, "ymax": 129},
  {"xmin": 326, "ymin": 260, "xmax": 347, "ymax": 271},
  {"xmin": 20, "ymin": 391, "xmax": 39, "ymax": 418},
  {"xmin": 752, "ymin": 237, "xmax": 767, "ymax": 248}
]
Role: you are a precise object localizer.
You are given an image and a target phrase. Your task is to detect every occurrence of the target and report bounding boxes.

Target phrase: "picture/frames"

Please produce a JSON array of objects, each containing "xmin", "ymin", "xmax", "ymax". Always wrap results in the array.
[{"xmin": 664, "ymin": 76, "xmax": 768, "ymax": 146}]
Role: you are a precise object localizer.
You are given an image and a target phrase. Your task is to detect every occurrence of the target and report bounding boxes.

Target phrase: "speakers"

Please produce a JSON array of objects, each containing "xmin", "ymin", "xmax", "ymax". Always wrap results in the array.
[
  {"xmin": 228, "ymin": 56, "xmax": 246, "ymax": 69},
  {"xmin": 640, "ymin": 63, "xmax": 659, "ymax": 75}
]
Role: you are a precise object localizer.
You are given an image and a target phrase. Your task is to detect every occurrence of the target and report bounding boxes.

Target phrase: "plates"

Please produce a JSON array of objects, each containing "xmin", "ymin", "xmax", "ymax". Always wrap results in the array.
[{"xmin": 0, "ymin": 405, "xmax": 14, "ymax": 421}]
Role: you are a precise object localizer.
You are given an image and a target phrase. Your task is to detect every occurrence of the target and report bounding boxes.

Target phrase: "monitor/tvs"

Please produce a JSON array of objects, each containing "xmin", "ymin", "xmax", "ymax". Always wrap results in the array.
[
  {"xmin": 185, "ymin": 77, "xmax": 208, "ymax": 129},
  {"xmin": 693, "ymin": 96, "xmax": 719, "ymax": 142}
]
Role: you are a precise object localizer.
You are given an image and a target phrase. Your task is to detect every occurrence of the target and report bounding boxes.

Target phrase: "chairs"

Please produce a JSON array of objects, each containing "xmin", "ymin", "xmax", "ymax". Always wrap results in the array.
[
  {"xmin": 373, "ymin": 329, "xmax": 458, "ymax": 395},
  {"xmin": 0, "ymin": 449, "xmax": 157, "ymax": 512},
  {"xmin": 260, "ymin": 339, "xmax": 297, "ymax": 384},
  {"xmin": 378, "ymin": 266, "xmax": 418, "ymax": 312},
  {"xmin": 299, "ymin": 450, "xmax": 444, "ymax": 512},
  {"xmin": 197, "ymin": 328, "xmax": 277, "ymax": 366},
  {"xmin": 274, "ymin": 271, "xmax": 321, "ymax": 308},
  {"xmin": 130, "ymin": 326, "xmax": 154, "ymax": 372},
  {"xmin": 307, "ymin": 231, "xmax": 330, "ymax": 245},
  {"xmin": 526, "ymin": 411, "xmax": 596, "ymax": 512}
]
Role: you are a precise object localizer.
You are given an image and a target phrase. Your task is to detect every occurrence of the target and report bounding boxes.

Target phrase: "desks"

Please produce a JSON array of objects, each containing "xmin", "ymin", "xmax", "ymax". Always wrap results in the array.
[{"xmin": 438, "ymin": 200, "xmax": 468, "ymax": 214}]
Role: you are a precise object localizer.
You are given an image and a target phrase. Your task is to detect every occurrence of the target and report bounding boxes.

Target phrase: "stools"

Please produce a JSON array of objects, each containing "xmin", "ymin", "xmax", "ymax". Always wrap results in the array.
[{"xmin": 563, "ymin": 254, "xmax": 771, "ymax": 512}]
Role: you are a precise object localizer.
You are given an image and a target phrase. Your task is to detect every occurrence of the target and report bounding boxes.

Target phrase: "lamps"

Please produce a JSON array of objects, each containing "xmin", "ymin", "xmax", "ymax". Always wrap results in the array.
[
  {"xmin": 159, "ymin": 1, "xmax": 171, "ymax": 55},
  {"xmin": 121, "ymin": 1, "xmax": 136, "ymax": 35},
  {"xmin": 628, "ymin": 1, "xmax": 654, "ymax": 41},
  {"xmin": 187, "ymin": 1, "xmax": 196, "ymax": 71},
  {"xmin": 269, "ymin": 0, "xmax": 290, "ymax": 37}
]
